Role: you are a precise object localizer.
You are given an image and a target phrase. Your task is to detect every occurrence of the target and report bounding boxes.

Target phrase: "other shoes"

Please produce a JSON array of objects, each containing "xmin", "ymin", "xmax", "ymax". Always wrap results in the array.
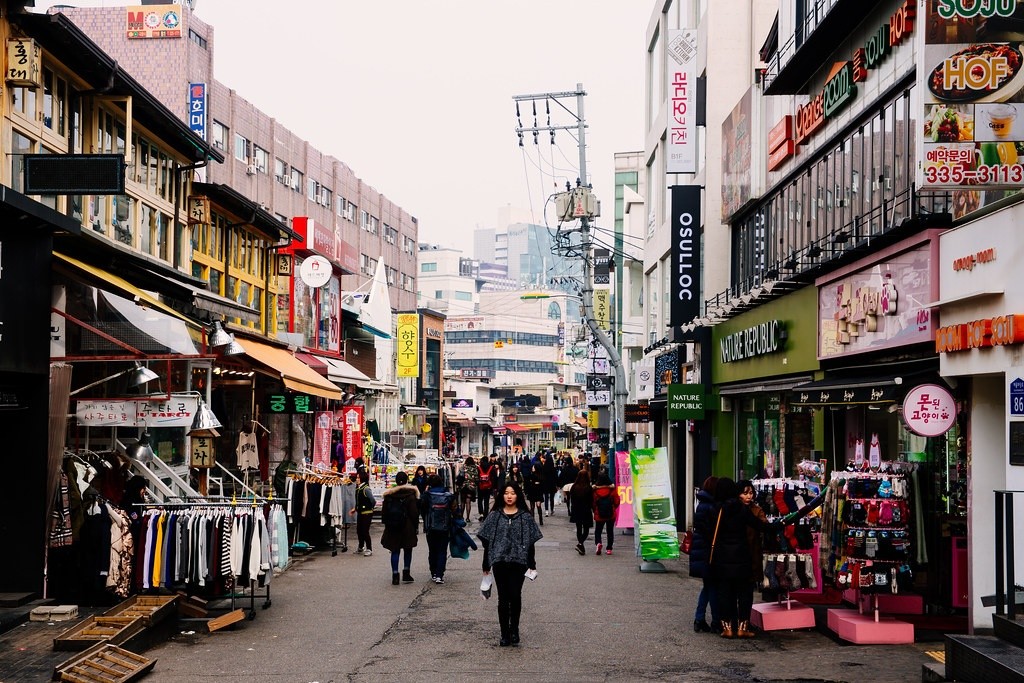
[
  {"xmin": 545, "ymin": 510, "xmax": 549, "ymax": 517},
  {"xmin": 596, "ymin": 543, "xmax": 603, "ymax": 555},
  {"xmin": 551, "ymin": 511, "xmax": 555, "ymax": 515},
  {"xmin": 606, "ymin": 550, "xmax": 612, "ymax": 555},
  {"xmin": 500, "ymin": 632, "xmax": 510, "ymax": 645},
  {"xmin": 353, "ymin": 547, "xmax": 365, "ymax": 554},
  {"xmin": 575, "ymin": 544, "xmax": 585, "ymax": 555},
  {"xmin": 436, "ymin": 576, "xmax": 444, "ymax": 584},
  {"xmin": 466, "ymin": 518, "xmax": 470, "ymax": 522},
  {"xmin": 432, "ymin": 573, "xmax": 436, "ymax": 581},
  {"xmin": 511, "ymin": 632, "xmax": 520, "ymax": 645},
  {"xmin": 364, "ymin": 549, "xmax": 372, "ymax": 556},
  {"xmin": 478, "ymin": 514, "xmax": 483, "ymax": 521}
]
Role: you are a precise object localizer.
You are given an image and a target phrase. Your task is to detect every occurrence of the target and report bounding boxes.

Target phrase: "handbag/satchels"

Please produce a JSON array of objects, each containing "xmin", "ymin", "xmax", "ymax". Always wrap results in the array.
[
  {"xmin": 457, "ymin": 465, "xmax": 465, "ymax": 486},
  {"xmin": 680, "ymin": 530, "xmax": 693, "ymax": 553},
  {"xmin": 450, "ymin": 539, "xmax": 469, "ymax": 559}
]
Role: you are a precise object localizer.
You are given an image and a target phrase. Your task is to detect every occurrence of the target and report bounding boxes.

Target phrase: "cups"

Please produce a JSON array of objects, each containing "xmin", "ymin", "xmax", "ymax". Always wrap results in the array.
[{"xmin": 986, "ymin": 104, "xmax": 1015, "ymax": 138}]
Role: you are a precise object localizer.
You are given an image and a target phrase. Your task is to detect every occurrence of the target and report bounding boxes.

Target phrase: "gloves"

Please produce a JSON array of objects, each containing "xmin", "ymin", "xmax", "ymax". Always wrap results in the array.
[{"xmin": 836, "ymin": 478, "xmax": 912, "ymax": 593}]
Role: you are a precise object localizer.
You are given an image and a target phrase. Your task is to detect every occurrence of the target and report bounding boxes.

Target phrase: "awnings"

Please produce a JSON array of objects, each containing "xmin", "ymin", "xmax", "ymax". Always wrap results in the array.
[
  {"xmin": 54, "ymin": 219, "xmax": 261, "ymax": 336},
  {"xmin": 448, "ymin": 419, "xmax": 475, "ymax": 426},
  {"xmin": 312, "ymin": 355, "xmax": 370, "ymax": 385},
  {"xmin": 403, "ymin": 405, "xmax": 430, "ymax": 415},
  {"xmin": 505, "ymin": 422, "xmax": 559, "ymax": 432},
  {"xmin": 229, "ymin": 336, "xmax": 342, "ymax": 400}
]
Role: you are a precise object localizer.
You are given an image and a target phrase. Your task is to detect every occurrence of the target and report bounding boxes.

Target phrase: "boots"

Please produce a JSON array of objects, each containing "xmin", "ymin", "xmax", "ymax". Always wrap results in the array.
[
  {"xmin": 721, "ymin": 620, "xmax": 734, "ymax": 638},
  {"xmin": 537, "ymin": 506, "xmax": 543, "ymax": 525},
  {"xmin": 737, "ymin": 619, "xmax": 756, "ymax": 637},
  {"xmin": 403, "ymin": 569, "xmax": 414, "ymax": 583},
  {"xmin": 392, "ymin": 572, "xmax": 400, "ymax": 585}
]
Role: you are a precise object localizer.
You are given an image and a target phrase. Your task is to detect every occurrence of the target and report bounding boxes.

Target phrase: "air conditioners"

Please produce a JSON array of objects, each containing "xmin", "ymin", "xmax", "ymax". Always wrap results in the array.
[
  {"xmin": 366, "ymin": 224, "xmax": 374, "ymax": 233},
  {"xmin": 283, "ymin": 175, "xmax": 295, "ymax": 189},
  {"xmin": 404, "ymin": 245, "xmax": 408, "ymax": 252},
  {"xmin": 386, "ymin": 235, "xmax": 393, "ymax": 244},
  {"xmin": 315, "ymin": 195, "xmax": 326, "ymax": 206},
  {"xmin": 342, "ymin": 210, "xmax": 351, "ymax": 220},
  {"xmin": 247, "ymin": 157, "xmax": 259, "ymax": 174},
  {"xmin": 341, "ymin": 290, "xmax": 364, "ymax": 310},
  {"xmin": 366, "ymin": 267, "xmax": 410, "ymax": 290}
]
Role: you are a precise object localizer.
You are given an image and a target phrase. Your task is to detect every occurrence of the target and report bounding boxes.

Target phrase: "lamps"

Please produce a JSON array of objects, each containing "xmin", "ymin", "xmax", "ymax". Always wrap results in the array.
[
  {"xmin": 644, "ymin": 336, "xmax": 672, "ymax": 357},
  {"xmin": 681, "ymin": 233, "xmax": 879, "ymax": 333},
  {"xmin": 130, "ymin": 361, "xmax": 159, "ymax": 388},
  {"xmin": 211, "ymin": 363, "xmax": 254, "ymax": 378},
  {"xmin": 66, "ymin": 448, "xmax": 112, "ymax": 477},
  {"xmin": 224, "ymin": 333, "xmax": 246, "ymax": 355},
  {"xmin": 178, "ymin": 310, "xmax": 233, "ymax": 347},
  {"xmin": 126, "ymin": 418, "xmax": 153, "ymax": 462},
  {"xmin": 166, "ymin": 391, "xmax": 222, "ymax": 430}
]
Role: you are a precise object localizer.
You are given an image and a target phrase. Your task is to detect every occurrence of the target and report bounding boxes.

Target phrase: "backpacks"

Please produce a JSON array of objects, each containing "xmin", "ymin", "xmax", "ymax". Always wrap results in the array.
[
  {"xmin": 478, "ymin": 466, "xmax": 493, "ymax": 490},
  {"xmin": 595, "ymin": 489, "xmax": 613, "ymax": 518},
  {"xmin": 425, "ymin": 492, "xmax": 451, "ymax": 531}
]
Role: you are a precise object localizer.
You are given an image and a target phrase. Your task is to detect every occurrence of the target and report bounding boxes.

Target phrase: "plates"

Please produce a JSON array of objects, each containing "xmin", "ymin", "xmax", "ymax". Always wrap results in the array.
[
  {"xmin": 952, "ymin": 190, "xmax": 985, "ymax": 218},
  {"xmin": 927, "ymin": 44, "xmax": 1023, "ymax": 101}
]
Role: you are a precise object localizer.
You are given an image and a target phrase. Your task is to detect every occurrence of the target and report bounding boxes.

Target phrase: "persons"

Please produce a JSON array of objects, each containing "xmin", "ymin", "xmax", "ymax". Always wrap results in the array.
[
  {"xmin": 332, "ymin": 435, "xmax": 345, "ymax": 472},
  {"xmin": 418, "ymin": 475, "xmax": 463, "ymax": 583},
  {"xmin": 354, "ymin": 457, "xmax": 369, "ymax": 471},
  {"xmin": 569, "ymin": 469, "xmax": 593, "ymax": 555},
  {"xmin": 592, "ymin": 472, "xmax": 620, "ymax": 555},
  {"xmin": 350, "ymin": 471, "xmax": 376, "ymax": 556},
  {"xmin": 690, "ymin": 476, "xmax": 784, "ymax": 637},
  {"xmin": 476, "ymin": 481, "xmax": 543, "ymax": 646},
  {"xmin": 117, "ymin": 475, "xmax": 148, "ymax": 515},
  {"xmin": 459, "ymin": 452, "xmax": 506, "ymax": 522},
  {"xmin": 556, "ymin": 453, "xmax": 607, "ymax": 515},
  {"xmin": 380, "ymin": 465, "xmax": 427, "ymax": 586},
  {"xmin": 505, "ymin": 451, "xmax": 559, "ymax": 525}
]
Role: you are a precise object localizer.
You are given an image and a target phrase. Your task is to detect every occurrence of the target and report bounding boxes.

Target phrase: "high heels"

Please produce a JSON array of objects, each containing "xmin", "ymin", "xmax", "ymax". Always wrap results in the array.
[
  {"xmin": 711, "ymin": 621, "xmax": 722, "ymax": 632},
  {"xmin": 694, "ymin": 618, "xmax": 711, "ymax": 632}
]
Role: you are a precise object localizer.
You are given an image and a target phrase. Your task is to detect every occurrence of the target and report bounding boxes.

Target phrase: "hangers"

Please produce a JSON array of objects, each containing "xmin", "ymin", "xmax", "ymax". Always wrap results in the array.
[
  {"xmin": 286, "ymin": 466, "xmax": 353, "ymax": 486},
  {"xmin": 141, "ymin": 496, "xmax": 284, "ymax": 516}
]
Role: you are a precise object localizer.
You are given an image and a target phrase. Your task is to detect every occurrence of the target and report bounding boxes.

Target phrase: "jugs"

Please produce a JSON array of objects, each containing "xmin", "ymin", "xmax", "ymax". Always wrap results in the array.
[{"xmin": 951, "ymin": 143, "xmax": 1018, "ymax": 181}]
